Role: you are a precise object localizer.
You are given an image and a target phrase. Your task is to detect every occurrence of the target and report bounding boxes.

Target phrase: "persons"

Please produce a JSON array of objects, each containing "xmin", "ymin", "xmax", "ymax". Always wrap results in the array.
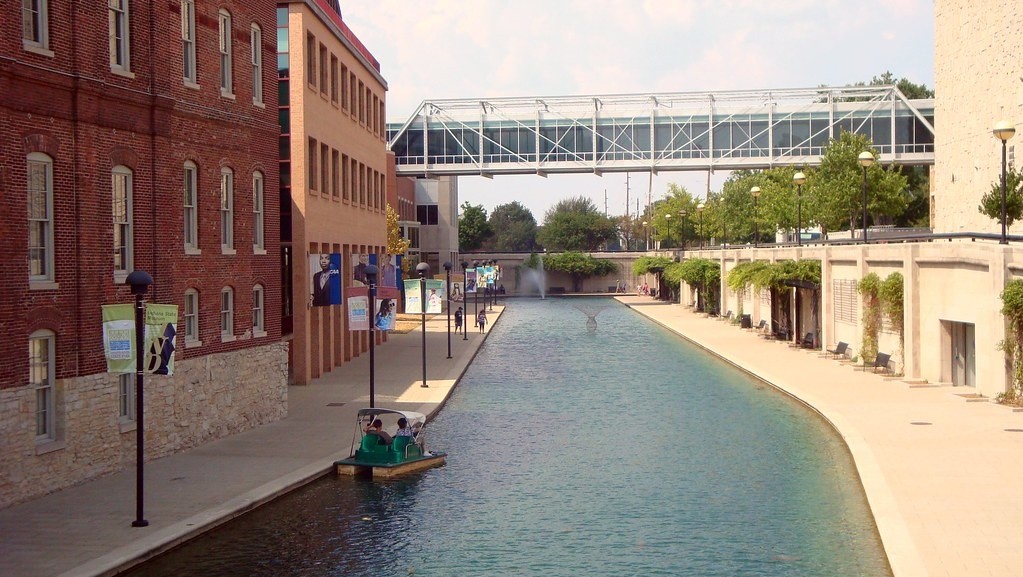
[
  {"xmin": 373, "ymin": 299, "xmax": 396, "ymax": 330},
  {"xmin": 616, "ymin": 279, "xmax": 649, "ymax": 297},
  {"xmin": 425, "ymin": 289, "xmax": 441, "ymax": 313},
  {"xmin": 454, "ymin": 307, "xmax": 463, "ymax": 335},
  {"xmin": 312, "ymin": 254, "xmax": 330, "ymax": 307},
  {"xmin": 477, "ymin": 310, "xmax": 488, "ymax": 334},
  {"xmin": 396, "ymin": 418, "xmax": 432, "ymax": 456},
  {"xmin": 380, "ymin": 254, "xmax": 396, "ymax": 286},
  {"xmin": 363, "ymin": 419, "xmax": 393, "ymax": 445},
  {"xmin": 354, "ymin": 254, "xmax": 369, "ymax": 282}
]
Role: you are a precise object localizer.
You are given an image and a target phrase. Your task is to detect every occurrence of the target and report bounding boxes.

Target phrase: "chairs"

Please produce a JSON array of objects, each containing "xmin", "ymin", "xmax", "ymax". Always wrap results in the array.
[
  {"xmin": 392, "ymin": 436, "xmax": 412, "ymax": 451},
  {"xmin": 362, "ymin": 436, "xmax": 381, "ymax": 451}
]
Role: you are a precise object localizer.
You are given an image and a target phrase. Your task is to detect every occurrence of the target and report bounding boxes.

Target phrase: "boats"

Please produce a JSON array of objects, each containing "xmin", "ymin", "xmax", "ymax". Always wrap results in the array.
[{"xmin": 332, "ymin": 407, "xmax": 448, "ymax": 477}]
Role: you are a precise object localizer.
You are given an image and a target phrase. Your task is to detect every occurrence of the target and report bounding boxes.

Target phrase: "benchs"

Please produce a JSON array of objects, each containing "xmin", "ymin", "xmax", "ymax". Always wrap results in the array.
[
  {"xmin": 550, "ymin": 286, "xmax": 565, "ymax": 293},
  {"xmin": 709, "ymin": 307, "xmax": 814, "ymax": 347},
  {"xmin": 608, "ymin": 286, "xmax": 618, "ymax": 292},
  {"xmin": 487, "ymin": 287, "xmax": 506, "ymax": 294},
  {"xmin": 861, "ymin": 351, "xmax": 891, "ymax": 374},
  {"xmin": 825, "ymin": 341, "xmax": 848, "ymax": 361}
]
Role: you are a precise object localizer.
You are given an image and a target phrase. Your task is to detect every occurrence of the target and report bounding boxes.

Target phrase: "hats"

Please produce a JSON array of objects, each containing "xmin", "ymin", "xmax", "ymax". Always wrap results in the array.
[{"xmin": 459, "ymin": 307, "xmax": 463, "ymax": 310}]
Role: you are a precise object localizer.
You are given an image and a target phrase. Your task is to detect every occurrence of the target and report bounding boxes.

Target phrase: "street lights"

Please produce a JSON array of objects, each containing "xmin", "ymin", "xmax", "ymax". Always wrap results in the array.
[
  {"xmin": 362, "ymin": 263, "xmax": 380, "ymax": 425},
  {"xmin": 793, "ymin": 172, "xmax": 807, "ymax": 246},
  {"xmin": 720, "ymin": 197, "xmax": 727, "ymax": 249},
  {"xmin": 750, "ymin": 185, "xmax": 761, "ymax": 248},
  {"xmin": 664, "ymin": 214, "xmax": 672, "ymax": 251},
  {"xmin": 493, "ymin": 258, "xmax": 498, "ymax": 305},
  {"xmin": 124, "ymin": 268, "xmax": 154, "ymax": 525},
  {"xmin": 992, "ymin": 119, "xmax": 1016, "ymax": 245},
  {"xmin": 482, "ymin": 260, "xmax": 488, "ymax": 314},
  {"xmin": 697, "ymin": 203, "xmax": 706, "ymax": 250},
  {"xmin": 415, "ymin": 261, "xmax": 430, "ymax": 388},
  {"xmin": 642, "ymin": 221, "xmax": 648, "ymax": 251},
  {"xmin": 461, "ymin": 261, "xmax": 470, "ymax": 340},
  {"xmin": 858, "ymin": 150, "xmax": 876, "ymax": 244},
  {"xmin": 472, "ymin": 259, "xmax": 479, "ymax": 327},
  {"xmin": 488, "ymin": 259, "xmax": 493, "ymax": 310},
  {"xmin": 442, "ymin": 260, "xmax": 454, "ymax": 360},
  {"xmin": 678, "ymin": 209, "xmax": 686, "ymax": 250}
]
